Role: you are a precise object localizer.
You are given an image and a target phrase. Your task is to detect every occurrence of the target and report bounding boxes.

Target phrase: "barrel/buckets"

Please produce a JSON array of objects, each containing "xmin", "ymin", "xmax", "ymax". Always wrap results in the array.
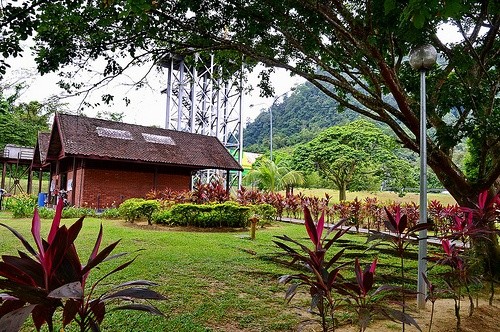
[{"xmin": 39, "ymin": 193, "xmax": 48, "ymax": 206}]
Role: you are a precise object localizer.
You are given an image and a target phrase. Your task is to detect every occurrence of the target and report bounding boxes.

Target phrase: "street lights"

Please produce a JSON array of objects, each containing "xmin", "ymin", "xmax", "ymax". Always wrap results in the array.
[
  {"xmin": 248, "ymin": 87, "xmax": 297, "ymax": 162},
  {"xmin": 405, "ymin": 42, "xmax": 439, "ymax": 314}
]
